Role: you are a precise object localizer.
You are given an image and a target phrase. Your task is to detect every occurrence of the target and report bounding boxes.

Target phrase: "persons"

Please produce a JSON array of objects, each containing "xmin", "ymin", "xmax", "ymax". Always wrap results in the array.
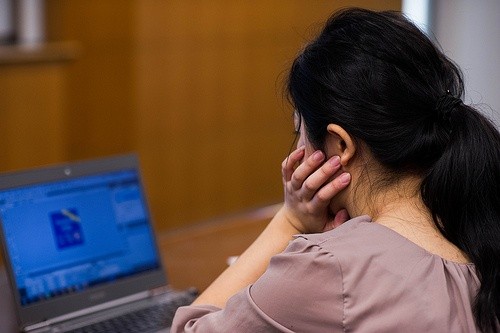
[{"xmin": 169, "ymin": 4, "xmax": 500, "ymax": 333}]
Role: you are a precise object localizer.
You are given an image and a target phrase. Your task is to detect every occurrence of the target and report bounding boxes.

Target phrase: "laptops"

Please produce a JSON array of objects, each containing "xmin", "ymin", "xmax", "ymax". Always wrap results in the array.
[{"xmin": 0, "ymin": 153, "xmax": 201, "ymax": 332}]
